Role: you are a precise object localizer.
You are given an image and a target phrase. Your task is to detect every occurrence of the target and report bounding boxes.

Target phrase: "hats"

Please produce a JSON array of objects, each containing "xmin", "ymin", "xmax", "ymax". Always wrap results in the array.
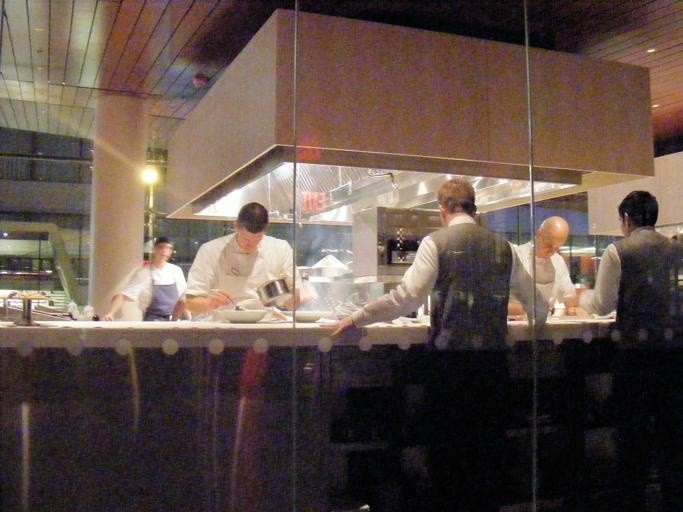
[{"xmin": 154, "ymin": 234, "xmax": 175, "ymax": 246}]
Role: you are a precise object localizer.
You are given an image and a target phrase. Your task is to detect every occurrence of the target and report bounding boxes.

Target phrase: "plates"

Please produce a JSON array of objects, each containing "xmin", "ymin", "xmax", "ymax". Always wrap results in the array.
[
  {"xmin": 288, "ymin": 310, "xmax": 320, "ymax": 321},
  {"xmin": 220, "ymin": 307, "xmax": 266, "ymax": 322}
]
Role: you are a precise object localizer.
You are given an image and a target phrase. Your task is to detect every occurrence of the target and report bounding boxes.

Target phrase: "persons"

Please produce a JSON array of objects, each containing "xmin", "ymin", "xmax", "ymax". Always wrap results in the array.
[
  {"xmin": 102, "ymin": 235, "xmax": 192, "ymax": 321},
  {"xmin": 574, "ymin": 188, "xmax": 682, "ymax": 509},
  {"xmin": 183, "ymin": 202, "xmax": 312, "ymax": 321},
  {"xmin": 505, "ymin": 215, "xmax": 589, "ymax": 322},
  {"xmin": 317, "ymin": 177, "xmax": 551, "ymax": 512}
]
